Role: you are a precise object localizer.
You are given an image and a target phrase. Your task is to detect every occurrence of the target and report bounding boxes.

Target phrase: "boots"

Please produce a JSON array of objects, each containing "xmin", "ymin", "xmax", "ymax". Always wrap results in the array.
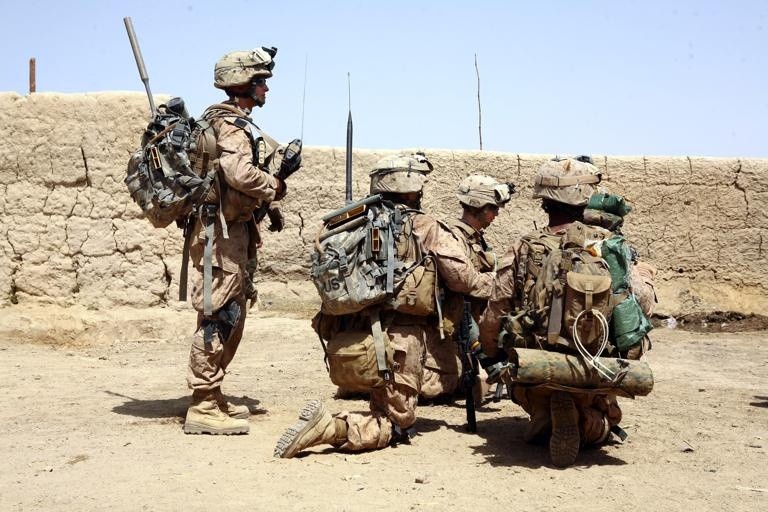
[
  {"xmin": 184, "ymin": 387, "xmax": 250, "ymax": 434},
  {"xmin": 274, "ymin": 397, "xmax": 349, "ymax": 458},
  {"xmin": 511, "ymin": 382, "xmax": 581, "ymax": 467}
]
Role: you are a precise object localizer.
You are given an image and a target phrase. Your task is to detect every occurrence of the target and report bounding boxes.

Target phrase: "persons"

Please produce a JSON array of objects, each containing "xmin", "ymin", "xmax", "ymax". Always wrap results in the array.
[
  {"xmin": 273, "ymin": 154, "xmax": 496, "ymax": 458},
  {"xmin": 181, "ymin": 42, "xmax": 300, "ymax": 435},
  {"xmin": 472, "ymin": 158, "xmax": 657, "ymax": 467},
  {"xmin": 419, "ymin": 171, "xmax": 512, "ymax": 402}
]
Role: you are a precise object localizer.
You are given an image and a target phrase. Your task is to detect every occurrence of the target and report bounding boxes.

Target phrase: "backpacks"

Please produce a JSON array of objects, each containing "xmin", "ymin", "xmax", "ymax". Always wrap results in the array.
[
  {"xmin": 313, "ymin": 193, "xmax": 424, "ymax": 314},
  {"xmin": 124, "ymin": 96, "xmax": 218, "ymax": 227},
  {"xmin": 519, "ymin": 247, "xmax": 611, "ymax": 353}
]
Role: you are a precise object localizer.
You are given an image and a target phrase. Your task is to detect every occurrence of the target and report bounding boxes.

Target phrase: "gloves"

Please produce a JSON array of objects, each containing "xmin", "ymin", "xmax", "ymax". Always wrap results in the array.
[{"xmin": 268, "ymin": 199, "xmax": 285, "ymax": 232}]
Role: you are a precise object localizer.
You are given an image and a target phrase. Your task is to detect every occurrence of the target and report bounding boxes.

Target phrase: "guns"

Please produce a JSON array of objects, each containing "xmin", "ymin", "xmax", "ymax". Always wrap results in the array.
[
  {"xmin": 255, "ymin": 138, "xmax": 302, "ymax": 222},
  {"xmin": 440, "ymin": 288, "xmax": 476, "ymax": 433}
]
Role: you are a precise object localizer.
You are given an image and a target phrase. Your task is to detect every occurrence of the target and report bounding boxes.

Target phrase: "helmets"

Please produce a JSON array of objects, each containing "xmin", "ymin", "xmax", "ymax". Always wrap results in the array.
[
  {"xmin": 369, "ymin": 154, "xmax": 433, "ymax": 193},
  {"xmin": 457, "ymin": 172, "xmax": 507, "ymax": 208},
  {"xmin": 214, "ymin": 49, "xmax": 273, "ymax": 89},
  {"xmin": 532, "ymin": 159, "xmax": 601, "ymax": 206}
]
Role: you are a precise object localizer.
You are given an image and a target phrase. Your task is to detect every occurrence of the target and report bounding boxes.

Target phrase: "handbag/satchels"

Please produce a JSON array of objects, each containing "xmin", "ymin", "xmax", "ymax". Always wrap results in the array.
[{"xmin": 326, "ymin": 331, "xmax": 392, "ymax": 392}]
